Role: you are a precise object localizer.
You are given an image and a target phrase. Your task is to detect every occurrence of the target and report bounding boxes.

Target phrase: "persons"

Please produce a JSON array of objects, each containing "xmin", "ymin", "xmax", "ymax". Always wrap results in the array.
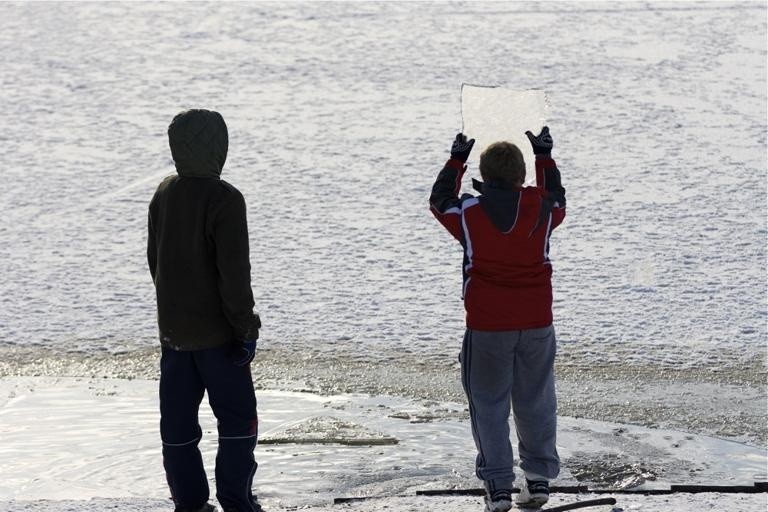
[
  {"xmin": 146, "ymin": 109, "xmax": 263, "ymax": 511},
  {"xmin": 426, "ymin": 125, "xmax": 568, "ymax": 512}
]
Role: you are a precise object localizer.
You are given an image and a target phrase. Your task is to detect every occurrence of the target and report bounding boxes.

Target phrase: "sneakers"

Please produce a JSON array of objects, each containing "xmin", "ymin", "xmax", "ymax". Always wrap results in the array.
[
  {"xmin": 514, "ymin": 478, "xmax": 551, "ymax": 508},
  {"xmin": 483, "ymin": 489, "xmax": 513, "ymax": 512},
  {"xmin": 196, "ymin": 503, "xmax": 218, "ymax": 512}
]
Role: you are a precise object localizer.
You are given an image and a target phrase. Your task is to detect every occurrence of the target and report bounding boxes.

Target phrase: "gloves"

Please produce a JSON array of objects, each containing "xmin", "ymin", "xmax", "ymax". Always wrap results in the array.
[
  {"xmin": 451, "ymin": 132, "xmax": 476, "ymax": 163},
  {"xmin": 525, "ymin": 124, "xmax": 554, "ymax": 157}
]
